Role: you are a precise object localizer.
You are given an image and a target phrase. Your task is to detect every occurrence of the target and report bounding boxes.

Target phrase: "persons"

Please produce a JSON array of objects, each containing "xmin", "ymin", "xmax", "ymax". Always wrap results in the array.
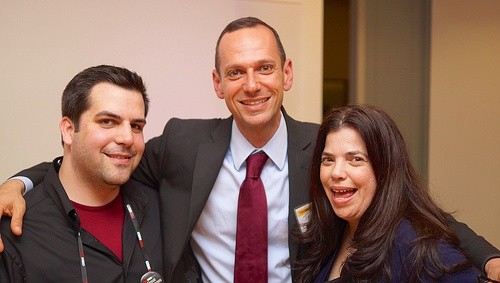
[
  {"xmin": 1, "ymin": 66, "xmax": 166, "ymax": 283},
  {"xmin": 0, "ymin": 15, "xmax": 500, "ymax": 283},
  {"xmin": 281, "ymin": 104, "xmax": 488, "ymax": 283}
]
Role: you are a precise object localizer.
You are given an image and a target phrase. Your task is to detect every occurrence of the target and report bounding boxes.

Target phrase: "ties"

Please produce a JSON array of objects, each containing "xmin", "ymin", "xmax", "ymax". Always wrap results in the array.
[{"xmin": 234, "ymin": 150, "xmax": 270, "ymax": 283}]
